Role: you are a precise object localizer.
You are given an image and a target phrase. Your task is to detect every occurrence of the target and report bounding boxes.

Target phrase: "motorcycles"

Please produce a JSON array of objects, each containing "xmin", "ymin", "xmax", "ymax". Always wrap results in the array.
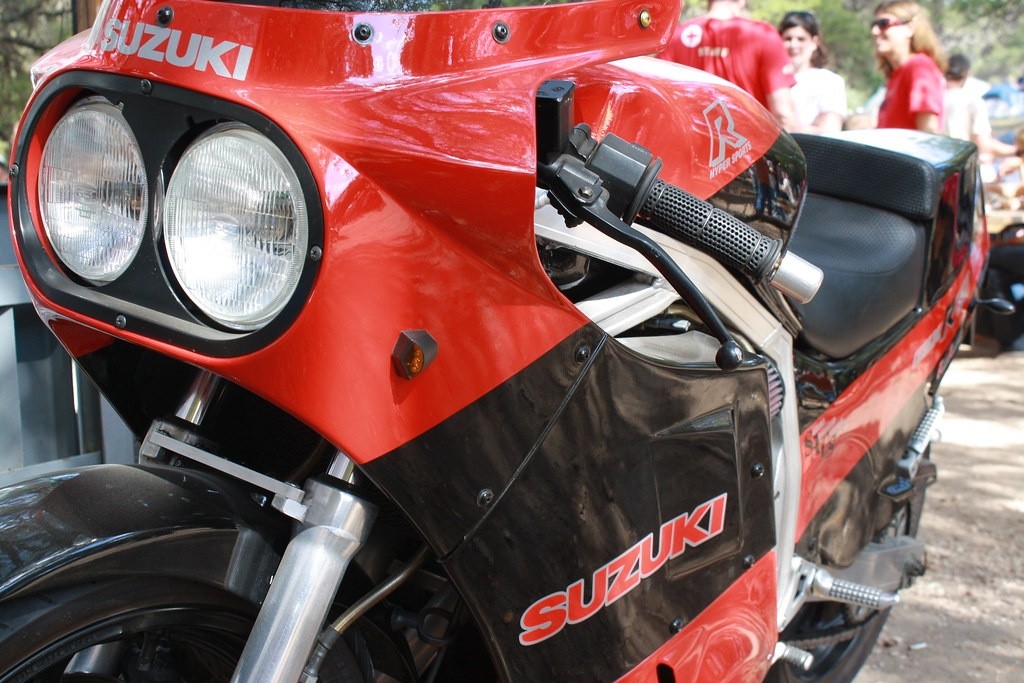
[{"xmin": 0, "ymin": 0, "xmax": 986, "ymax": 682}]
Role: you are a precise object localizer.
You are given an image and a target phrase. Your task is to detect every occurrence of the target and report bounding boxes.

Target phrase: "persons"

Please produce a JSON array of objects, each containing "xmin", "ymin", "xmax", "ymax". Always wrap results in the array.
[
  {"xmin": 0, "ymin": 153, "xmax": 11, "ymax": 185},
  {"xmin": 1012, "ymin": 75, "xmax": 1024, "ymax": 103},
  {"xmin": 769, "ymin": 10, "xmax": 847, "ymax": 132},
  {"xmin": 652, "ymin": 0, "xmax": 808, "ymax": 137},
  {"xmin": 869, "ymin": 1, "xmax": 947, "ymax": 145},
  {"xmin": 937, "ymin": 54, "xmax": 1020, "ymax": 173},
  {"xmin": 996, "ymin": 126, "xmax": 1024, "ymax": 177},
  {"xmin": 10, "ymin": 110, "xmax": 22, "ymax": 144}
]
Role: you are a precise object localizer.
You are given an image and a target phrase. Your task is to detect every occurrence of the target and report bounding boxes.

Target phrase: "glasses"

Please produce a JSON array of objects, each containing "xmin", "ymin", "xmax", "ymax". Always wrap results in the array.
[{"xmin": 871, "ymin": 18, "xmax": 914, "ymax": 31}]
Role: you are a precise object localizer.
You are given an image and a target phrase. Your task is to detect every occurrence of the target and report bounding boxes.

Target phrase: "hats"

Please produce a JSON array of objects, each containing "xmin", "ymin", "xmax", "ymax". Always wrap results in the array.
[{"xmin": 946, "ymin": 53, "xmax": 971, "ymax": 77}]
